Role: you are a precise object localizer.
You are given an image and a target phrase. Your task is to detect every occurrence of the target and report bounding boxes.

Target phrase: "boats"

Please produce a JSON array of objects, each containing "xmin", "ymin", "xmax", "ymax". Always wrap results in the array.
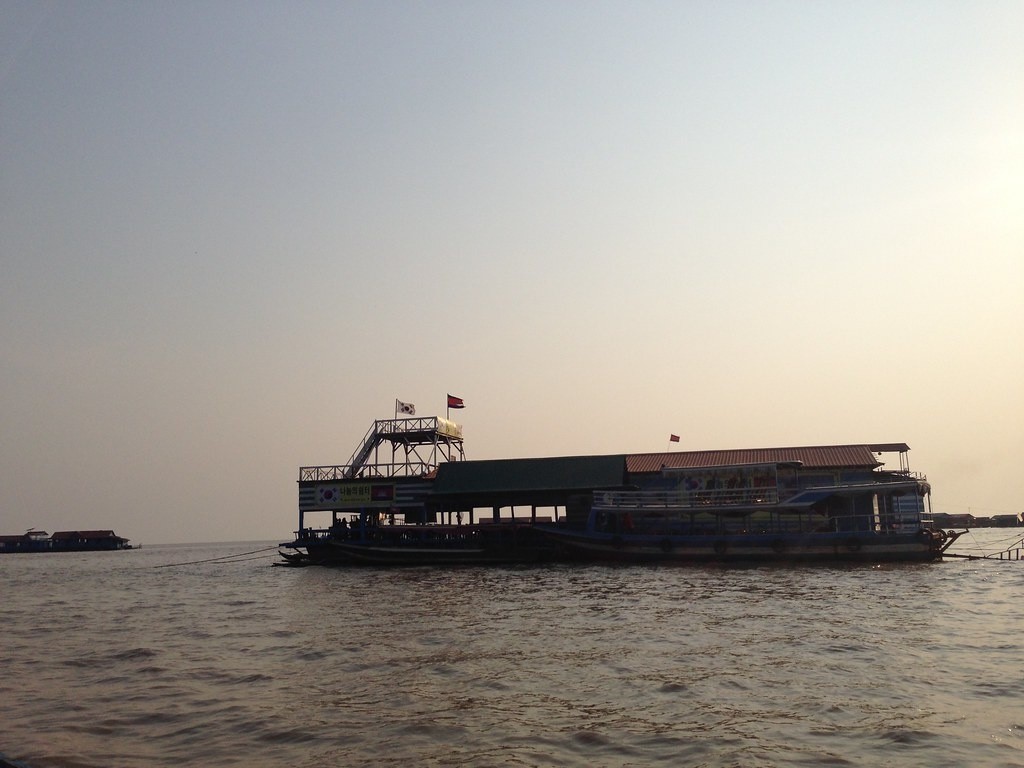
[{"xmin": 276, "ymin": 416, "xmax": 971, "ymax": 567}]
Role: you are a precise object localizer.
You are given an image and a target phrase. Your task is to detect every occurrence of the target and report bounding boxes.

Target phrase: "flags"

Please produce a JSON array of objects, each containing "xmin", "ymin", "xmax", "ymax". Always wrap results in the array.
[
  {"xmin": 397, "ymin": 400, "xmax": 415, "ymax": 415},
  {"xmin": 447, "ymin": 394, "xmax": 465, "ymax": 409},
  {"xmin": 670, "ymin": 434, "xmax": 680, "ymax": 442}
]
